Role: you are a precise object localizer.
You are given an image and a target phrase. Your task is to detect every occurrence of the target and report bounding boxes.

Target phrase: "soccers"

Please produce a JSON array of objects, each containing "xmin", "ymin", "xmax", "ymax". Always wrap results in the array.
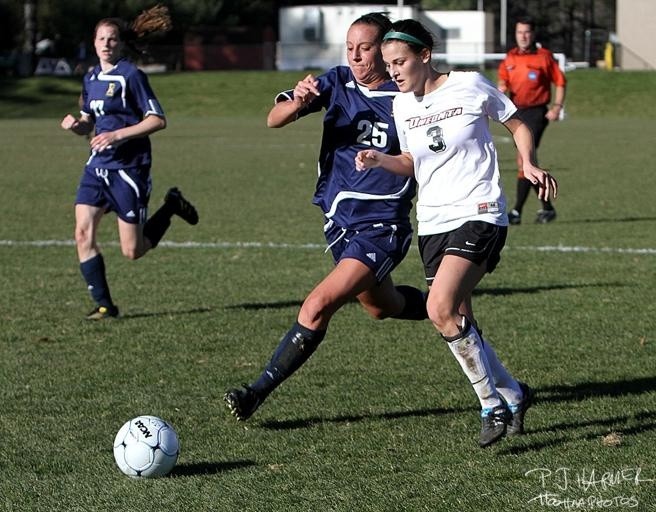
[{"xmin": 113, "ymin": 416, "xmax": 179, "ymax": 478}]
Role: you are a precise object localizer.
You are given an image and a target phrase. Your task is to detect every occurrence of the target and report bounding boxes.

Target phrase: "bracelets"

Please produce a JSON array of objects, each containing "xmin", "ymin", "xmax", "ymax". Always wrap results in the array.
[{"xmin": 554, "ymin": 104, "xmax": 563, "ymax": 109}]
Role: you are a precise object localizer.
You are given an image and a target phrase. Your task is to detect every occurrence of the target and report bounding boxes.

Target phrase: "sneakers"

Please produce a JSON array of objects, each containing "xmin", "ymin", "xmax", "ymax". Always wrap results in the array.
[
  {"xmin": 222, "ymin": 382, "xmax": 260, "ymax": 421},
  {"xmin": 165, "ymin": 187, "xmax": 199, "ymax": 228},
  {"xmin": 479, "ymin": 391, "xmax": 514, "ymax": 447},
  {"xmin": 88, "ymin": 305, "xmax": 120, "ymax": 319},
  {"xmin": 509, "ymin": 380, "xmax": 537, "ymax": 431},
  {"xmin": 536, "ymin": 209, "xmax": 557, "ymax": 222},
  {"xmin": 507, "ymin": 208, "xmax": 521, "ymax": 225}
]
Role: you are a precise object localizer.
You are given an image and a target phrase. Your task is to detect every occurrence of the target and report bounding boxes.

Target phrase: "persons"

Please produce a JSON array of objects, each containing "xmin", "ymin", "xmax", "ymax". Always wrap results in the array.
[
  {"xmin": 61, "ymin": 4, "xmax": 200, "ymax": 322},
  {"xmin": 354, "ymin": 21, "xmax": 559, "ymax": 447},
  {"xmin": 496, "ymin": 20, "xmax": 569, "ymax": 227},
  {"xmin": 224, "ymin": 11, "xmax": 432, "ymax": 425}
]
